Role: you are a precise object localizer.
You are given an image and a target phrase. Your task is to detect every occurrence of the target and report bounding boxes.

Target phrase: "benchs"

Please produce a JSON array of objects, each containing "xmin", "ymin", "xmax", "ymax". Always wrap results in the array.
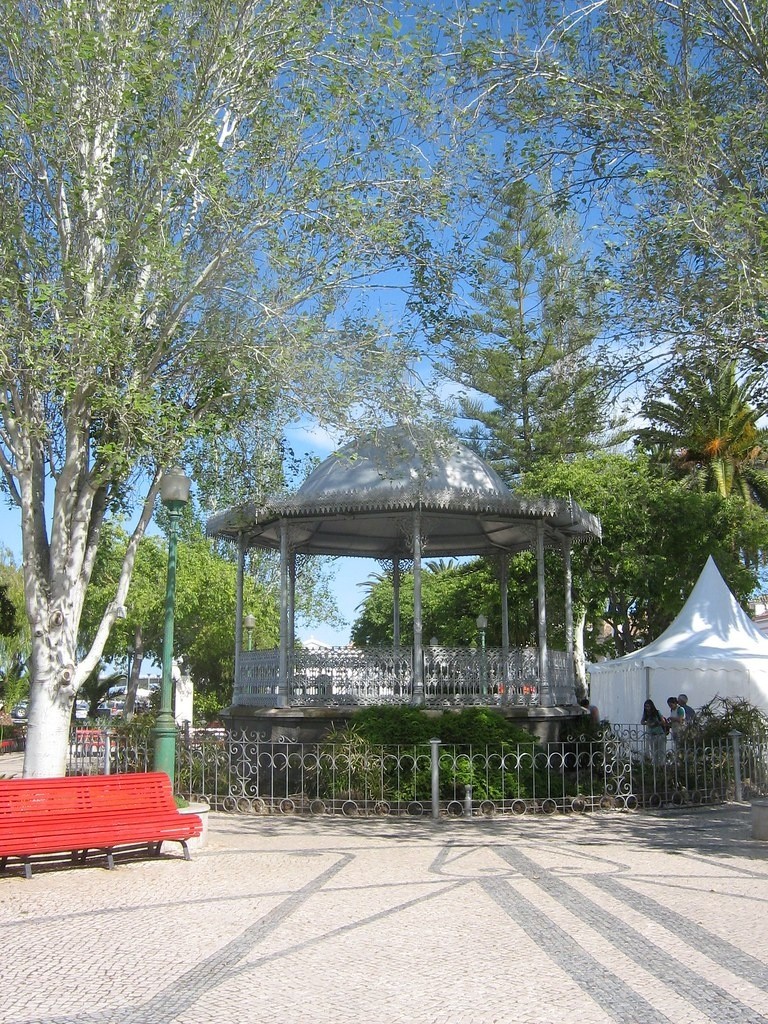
[
  {"xmin": 77, "ymin": 729, "xmax": 125, "ymax": 756},
  {"xmin": 0, "ymin": 771, "xmax": 203, "ymax": 879}
]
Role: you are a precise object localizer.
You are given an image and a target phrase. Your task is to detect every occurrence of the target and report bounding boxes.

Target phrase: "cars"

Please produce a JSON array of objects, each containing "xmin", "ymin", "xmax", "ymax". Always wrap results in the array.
[{"xmin": 10, "ymin": 699, "xmax": 30, "ymax": 719}]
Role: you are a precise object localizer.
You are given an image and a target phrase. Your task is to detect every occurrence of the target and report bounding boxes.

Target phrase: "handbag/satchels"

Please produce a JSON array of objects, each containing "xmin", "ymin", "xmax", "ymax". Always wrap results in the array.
[{"xmin": 657, "ymin": 709, "xmax": 670, "ymax": 735}]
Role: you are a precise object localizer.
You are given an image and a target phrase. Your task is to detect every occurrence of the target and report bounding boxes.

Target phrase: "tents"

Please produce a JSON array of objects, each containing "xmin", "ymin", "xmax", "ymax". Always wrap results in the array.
[{"xmin": 588, "ymin": 555, "xmax": 768, "ymax": 765}]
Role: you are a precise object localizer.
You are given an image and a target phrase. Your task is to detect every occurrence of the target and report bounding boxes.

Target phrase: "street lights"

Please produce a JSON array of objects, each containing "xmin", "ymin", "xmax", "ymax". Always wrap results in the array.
[
  {"xmin": 149, "ymin": 470, "xmax": 192, "ymax": 800},
  {"xmin": 475, "ymin": 613, "xmax": 489, "ymax": 695},
  {"xmin": 126, "ymin": 642, "xmax": 133, "ymax": 693},
  {"xmin": 245, "ymin": 613, "xmax": 255, "ymax": 694}
]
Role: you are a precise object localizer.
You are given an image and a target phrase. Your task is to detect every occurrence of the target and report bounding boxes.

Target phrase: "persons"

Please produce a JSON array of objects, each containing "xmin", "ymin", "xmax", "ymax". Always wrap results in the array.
[
  {"xmin": 134, "ymin": 700, "xmax": 148, "ymax": 714},
  {"xmin": 580, "ymin": 700, "xmax": 600, "ymax": 731},
  {"xmin": 497, "ymin": 672, "xmax": 536, "ymax": 706},
  {"xmin": 0, "ymin": 705, "xmax": 13, "ymax": 726},
  {"xmin": 641, "ymin": 699, "xmax": 666, "ymax": 766},
  {"xmin": 667, "ymin": 694, "xmax": 697, "ymax": 749}
]
